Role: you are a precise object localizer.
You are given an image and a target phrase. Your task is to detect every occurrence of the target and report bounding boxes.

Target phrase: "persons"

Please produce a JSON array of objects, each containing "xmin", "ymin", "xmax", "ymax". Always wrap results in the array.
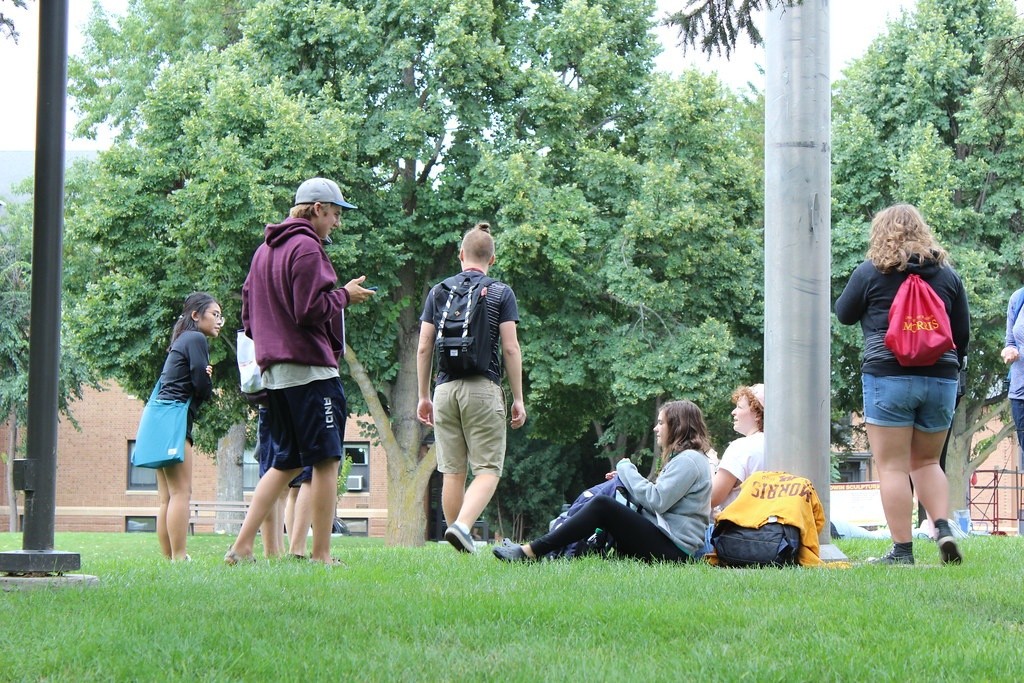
[
  {"xmin": 157, "ymin": 292, "xmax": 223, "ymax": 564},
  {"xmin": 832, "ymin": 203, "xmax": 971, "ymax": 565},
  {"xmin": 1001, "ymin": 286, "xmax": 1024, "ymax": 450},
  {"xmin": 908, "ymin": 370, "xmax": 967, "ymax": 541},
  {"xmin": 218, "ymin": 176, "xmax": 378, "ymax": 564},
  {"xmin": 414, "ymin": 220, "xmax": 526, "ymax": 551},
  {"xmin": 493, "ymin": 384, "xmax": 765, "ymax": 564}
]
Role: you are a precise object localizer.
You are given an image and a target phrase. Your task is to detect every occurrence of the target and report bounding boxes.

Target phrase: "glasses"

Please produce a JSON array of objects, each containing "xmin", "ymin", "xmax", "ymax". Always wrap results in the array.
[{"xmin": 204, "ymin": 311, "xmax": 225, "ymax": 327}]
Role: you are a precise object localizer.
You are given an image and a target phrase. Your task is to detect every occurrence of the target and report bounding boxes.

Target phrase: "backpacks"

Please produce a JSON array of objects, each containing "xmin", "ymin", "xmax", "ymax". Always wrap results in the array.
[
  {"xmin": 548, "ymin": 474, "xmax": 630, "ymax": 560},
  {"xmin": 435, "ymin": 277, "xmax": 498, "ymax": 378},
  {"xmin": 864, "ymin": 274, "xmax": 956, "ymax": 367},
  {"xmin": 712, "ymin": 521, "xmax": 800, "ymax": 567}
]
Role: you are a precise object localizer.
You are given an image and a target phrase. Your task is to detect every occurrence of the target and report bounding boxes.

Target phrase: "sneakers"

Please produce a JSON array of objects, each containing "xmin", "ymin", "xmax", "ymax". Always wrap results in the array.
[
  {"xmin": 866, "ymin": 542, "xmax": 914, "ymax": 564},
  {"xmin": 445, "ymin": 523, "xmax": 475, "ymax": 553},
  {"xmin": 931, "ymin": 519, "xmax": 963, "ymax": 564}
]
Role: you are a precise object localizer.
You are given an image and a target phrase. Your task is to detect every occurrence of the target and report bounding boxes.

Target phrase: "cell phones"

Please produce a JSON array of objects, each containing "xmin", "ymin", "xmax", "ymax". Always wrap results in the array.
[{"xmin": 368, "ymin": 286, "xmax": 378, "ymax": 291}]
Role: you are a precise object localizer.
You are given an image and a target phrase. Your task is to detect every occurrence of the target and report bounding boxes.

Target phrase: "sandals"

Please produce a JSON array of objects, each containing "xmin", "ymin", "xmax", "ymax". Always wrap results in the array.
[
  {"xmin": 502, "ymin": 537, "xmax": 515, "ymax": 545},
  {"xmin": 492, "ymin": 544, "xmax": 530, "ymax": 563},
  {"xmin": 224, "ymin": 549, "xmax": 256, "ymax": 565}
]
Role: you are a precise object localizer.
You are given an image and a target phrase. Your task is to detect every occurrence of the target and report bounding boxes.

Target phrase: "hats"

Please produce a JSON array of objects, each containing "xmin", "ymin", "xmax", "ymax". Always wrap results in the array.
[
  {"xmin": 749, "ymin": 383, "xmax": 765, "ymax": 409},
  {"xmin": 295, "ymin": 177, "xmax": 358, "ymax": 211}
]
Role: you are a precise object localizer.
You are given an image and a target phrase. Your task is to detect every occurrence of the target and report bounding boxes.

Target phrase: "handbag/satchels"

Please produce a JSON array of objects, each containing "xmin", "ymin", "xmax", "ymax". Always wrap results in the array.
[
  {"xmin": 134, "ymin": 399, "xmax": 188, "ymax": 469},
  {"xmin": 235, "ymin": 328, "xmax": 264, "ymax": 393}
]
